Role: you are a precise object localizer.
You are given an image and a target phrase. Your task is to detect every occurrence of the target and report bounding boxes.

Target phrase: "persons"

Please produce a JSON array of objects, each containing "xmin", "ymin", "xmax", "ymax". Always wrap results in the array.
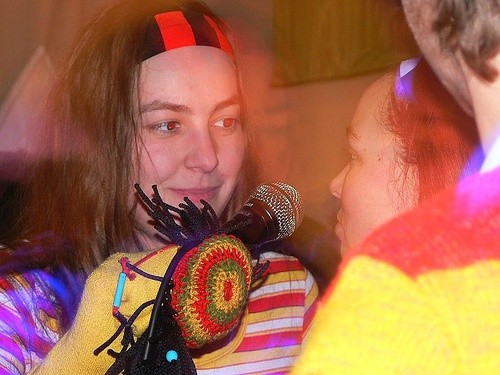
[
  {"xmin": 0, "ymin": 0, "xmax": 324, "ymax": 375},
  {"xmin": 328, "ymin": 56, "xmax": 487, "ymax": 271},
  {"xmin": 290, "ymin": 1, "xmax": 498, "ymax": 375}
]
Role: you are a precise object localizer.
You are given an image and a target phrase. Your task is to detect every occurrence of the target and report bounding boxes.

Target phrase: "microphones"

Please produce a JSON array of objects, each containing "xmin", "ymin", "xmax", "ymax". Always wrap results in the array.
[{"xmin": 206, "ymin": 182, "xmax": 305, "ymax": 254}]
